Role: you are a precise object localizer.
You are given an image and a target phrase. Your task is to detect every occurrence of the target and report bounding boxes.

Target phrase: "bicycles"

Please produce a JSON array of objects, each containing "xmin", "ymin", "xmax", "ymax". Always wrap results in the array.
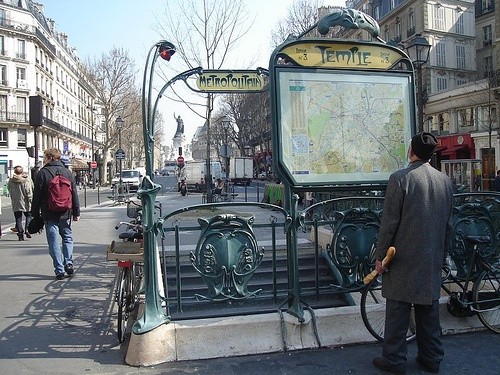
[
  {"xmin": 360, "ymin": 234, "xmax": 500, "ymax": 342},
  {"xmin": 116, "ymin": 201, "xmax": 164, "ymax": 342}
]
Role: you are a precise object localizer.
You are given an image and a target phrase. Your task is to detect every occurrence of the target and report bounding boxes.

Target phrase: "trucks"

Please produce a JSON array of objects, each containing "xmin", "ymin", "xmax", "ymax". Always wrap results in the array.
[{"xmin": 229, "ymin": 156, "xmax": 254, "ymax": 186}]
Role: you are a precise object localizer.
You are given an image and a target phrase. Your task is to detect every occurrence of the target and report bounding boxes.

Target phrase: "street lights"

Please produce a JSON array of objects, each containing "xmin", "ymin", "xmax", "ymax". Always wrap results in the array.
[
  {"xmin": 220, "ymin": 118, "xmax": 232, "ymax": 193},
  {"xmin": 115, "ymin": 116, "xmax": 125, "ymax": 202}
]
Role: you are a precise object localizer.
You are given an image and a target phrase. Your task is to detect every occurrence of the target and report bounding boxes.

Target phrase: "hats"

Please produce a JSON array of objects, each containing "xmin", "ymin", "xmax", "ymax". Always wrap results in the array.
[
  {"xmin": 412, "ymin": 132, "xmax": 439, "ymax": 161},
  {"xmin": 28, "ymin": 217, "xmax": 44, "ymax": 234}
]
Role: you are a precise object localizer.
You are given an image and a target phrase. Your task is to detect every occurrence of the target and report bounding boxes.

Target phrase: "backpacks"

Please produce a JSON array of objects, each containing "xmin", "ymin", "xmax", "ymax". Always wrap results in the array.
[{"xmin": 42, "ymin": 167, "xmax": 73, "ymax": 212}]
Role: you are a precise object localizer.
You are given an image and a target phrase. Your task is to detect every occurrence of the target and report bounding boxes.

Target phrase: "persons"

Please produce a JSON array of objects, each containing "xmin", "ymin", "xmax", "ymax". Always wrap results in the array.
[
  {"xmin": 495, "ymin": 170, "xmax": 500, "ymax": 192},
  {"xmin": 476, "ymin": 175, "xmax": 482, "ymax": 191},
  {"xmin": 31, "ymin": 148, "xmax": 80, "ymax": 280},
  {"xmin": 76, "ymin": 171, "xmax": 93, "ymax": 190},
  {"xmin": 212, "ymin": 178, "xmax": 224, "ymax": 194},
  {"xmin": 7, "ymin": 166, "xmax": 43, "ymax": 241},
  {"xmin": 372, "ymin": 132, "xmax": 454, "ymax": 375}
]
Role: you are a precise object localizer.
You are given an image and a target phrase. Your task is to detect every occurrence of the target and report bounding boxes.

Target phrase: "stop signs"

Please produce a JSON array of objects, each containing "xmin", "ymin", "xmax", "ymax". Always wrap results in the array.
[{"xmin": 178, "ymin": 157, "xmax": 184, "ymax": 162}]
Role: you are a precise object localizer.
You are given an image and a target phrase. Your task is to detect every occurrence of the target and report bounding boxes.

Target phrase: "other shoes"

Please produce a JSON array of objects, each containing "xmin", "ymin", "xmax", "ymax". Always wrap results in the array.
[
  {"xmin": 64, "ymin": 264, "xmax": 74, "ymax": 274},
  {"xmin": 24, "ymin": 230, "xmax": 31, "ymax": 238},
  {"xmin": 56, "ymin": 271, "xmax": 68, "ymax": 280},
  {"xmin": 416, "ymin": 357, "xmax": 439, "ymax": 373},
  {"xmin": 80, "ymin": 188, "xmax": 81, "ymax": 190},
  {"xmin": 17, "ymin": 233, "xmax": 24, "ymax": 241},
  {"xmin": 373, "ymin": 357, "xmax": 406, "ymax": 373}
]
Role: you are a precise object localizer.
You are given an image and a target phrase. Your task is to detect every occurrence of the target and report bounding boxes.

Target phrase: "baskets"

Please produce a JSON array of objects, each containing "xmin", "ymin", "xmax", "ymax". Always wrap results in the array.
[{"xmin": 127, "ymin": 201, "xmax": 142, "ymax": 218}]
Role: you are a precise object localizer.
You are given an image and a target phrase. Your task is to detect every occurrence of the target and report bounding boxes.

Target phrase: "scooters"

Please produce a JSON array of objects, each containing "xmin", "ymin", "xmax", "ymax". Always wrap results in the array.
[{"xmin": 179, "ymin": 178, "xmax": 187, "ymax": 196}]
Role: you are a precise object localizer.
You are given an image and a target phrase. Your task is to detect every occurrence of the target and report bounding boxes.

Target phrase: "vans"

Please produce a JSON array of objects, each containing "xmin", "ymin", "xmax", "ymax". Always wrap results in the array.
[{"xmin": 178, "ymin": 163, "xmax": 222, "ymax": 193}]
